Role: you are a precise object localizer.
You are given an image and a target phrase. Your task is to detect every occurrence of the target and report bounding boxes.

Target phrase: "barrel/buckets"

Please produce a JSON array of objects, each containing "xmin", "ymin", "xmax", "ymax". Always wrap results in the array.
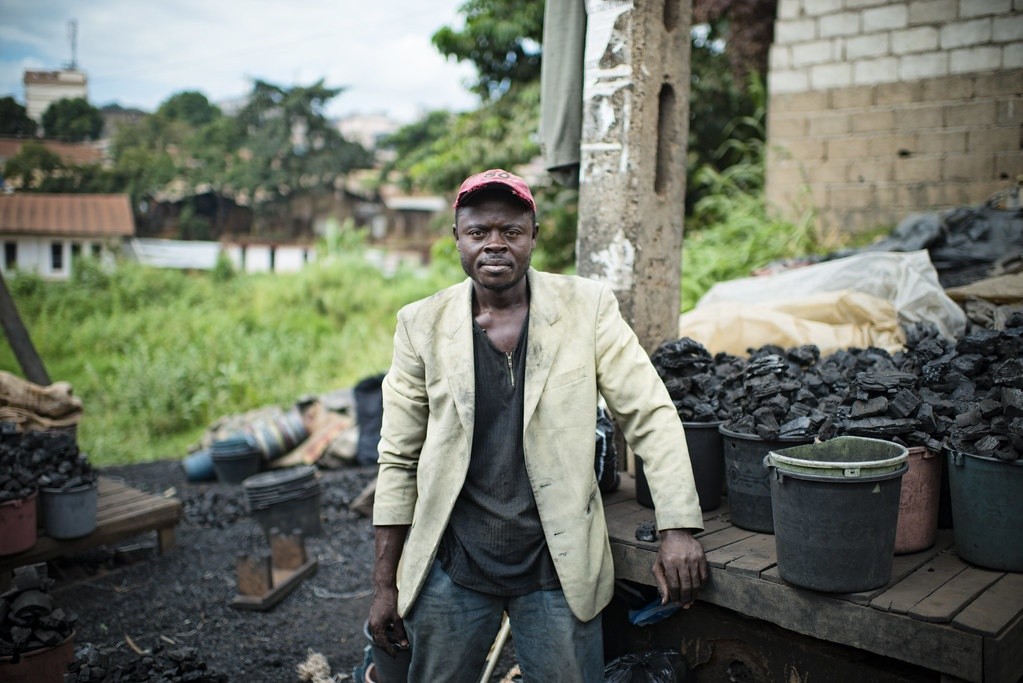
[
  {"xmin": 363, "ymin": 618, "xmax": 412, "ymax": 682},
  {"xmin": 39, "ymin": 476, "xmax": 99, "ymax": 539},
  {"xmin": 630, "ymin": 415, "xmax": 1023, "ymax": 591},
  {"xmin": 0, "ymin": 629, "xmax": 79, "ymax": 683},
  {"xmin": 181, "ymin": 373, "xmax": 385, "ymax": 538},
  {"xmin": 1, "ymin": 489, "xmax": 37, "ymax": 553}
]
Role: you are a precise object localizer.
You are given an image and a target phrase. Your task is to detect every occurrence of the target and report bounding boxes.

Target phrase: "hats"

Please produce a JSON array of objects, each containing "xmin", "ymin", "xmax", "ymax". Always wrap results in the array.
[{"xmin": 454, "ymin": 168, "xmax": 537, "ymax": 213}]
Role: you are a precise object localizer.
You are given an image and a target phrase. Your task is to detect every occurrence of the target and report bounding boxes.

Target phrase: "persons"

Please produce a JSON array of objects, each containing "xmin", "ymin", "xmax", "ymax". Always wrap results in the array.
[{"xmin": 367, "ymin": 170, "xmax": 710, "ymax": 683}]
